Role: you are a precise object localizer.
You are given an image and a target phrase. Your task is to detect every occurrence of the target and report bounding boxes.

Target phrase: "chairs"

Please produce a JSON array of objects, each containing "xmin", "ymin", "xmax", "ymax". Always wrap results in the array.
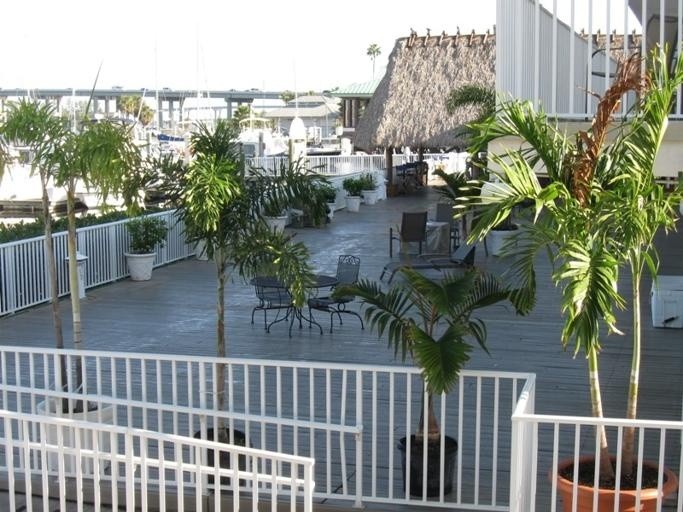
[{"xmin": 245, "ymin": 196, "xmax": 480, "ymax": 342}]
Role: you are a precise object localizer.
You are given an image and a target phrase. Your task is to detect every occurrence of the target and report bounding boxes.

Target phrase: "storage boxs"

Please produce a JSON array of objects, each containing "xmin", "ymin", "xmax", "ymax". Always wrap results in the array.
[{"xmin": 648, "ymin": 274, "xmax": 682, "ymax": 329}]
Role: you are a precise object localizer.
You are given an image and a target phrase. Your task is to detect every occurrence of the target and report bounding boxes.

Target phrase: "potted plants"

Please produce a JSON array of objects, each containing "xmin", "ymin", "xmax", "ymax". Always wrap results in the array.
[
  {"xmin": 440, "ymin": 36, "xmax": 682, "ymax": 512},
  {"xmin": 428, "ymin": 151, "xmax": 535, "ymax": 258},
  {"xmin": 0, "ymin": 95, "xmax": 149, "ymax": 475},
  {"xmin": 235, "ymin": 147, "xmax": 382, "ymax": 239},
  {"xmin": 328, "ymin": 246, "xmax": 554, "ymax": 495},
  {"xmin": 147, "ymin": 99, "xmax": 274, "ymax": 497},
  {"xmin": 122, "ymin": 215, "xmax": 171, "ymax": 282}
]
